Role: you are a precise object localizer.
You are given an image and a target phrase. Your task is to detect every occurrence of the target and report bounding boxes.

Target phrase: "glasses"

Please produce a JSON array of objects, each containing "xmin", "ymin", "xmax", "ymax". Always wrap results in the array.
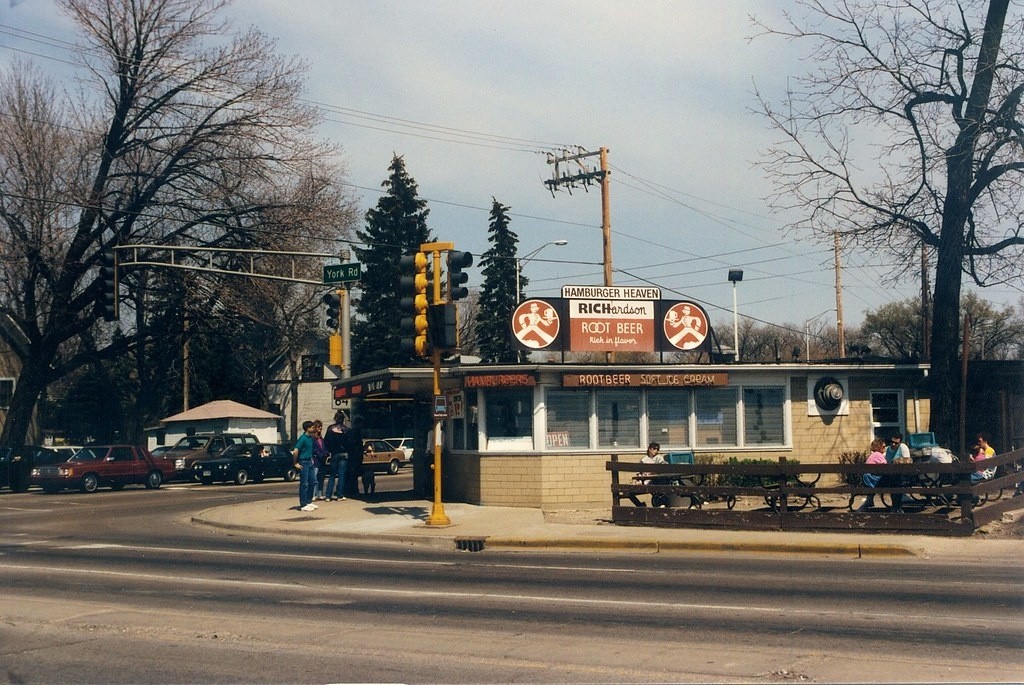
[
  {"xmin": 652, "ymin": 447, "xmax": 659, "ymax": 451},
  {"xmin": 892, "ymin": 440, "xmax": 900, "ymax": 444}
]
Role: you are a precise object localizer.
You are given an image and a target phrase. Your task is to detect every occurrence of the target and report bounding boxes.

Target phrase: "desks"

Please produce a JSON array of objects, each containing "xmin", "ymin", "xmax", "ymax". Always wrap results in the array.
[
  {"xmin": 757, "ymin": 470, "xmax": 822, "ymax": 512},
  {"xmin": 631, "ymin": 473, "xmax": 705, "ymax": 509},
  {"xmin": 855, "ymin": 472, "xmax": 935, "ymax": 512}
]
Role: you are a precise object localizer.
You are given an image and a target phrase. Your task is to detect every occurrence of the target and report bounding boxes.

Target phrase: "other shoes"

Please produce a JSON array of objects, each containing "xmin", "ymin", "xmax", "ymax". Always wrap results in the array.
[
  {"xmin": 337, "ymin": 498, "xmax": 343, "ymax": 501},
  {"xmin": 312, "ymin": 496, "xmax": 318, "ymax": 500},
  {"xmin": 319, "ymin": 495, "xmax": 326, "ymax": 499},
  {"xmin": 326, "ymin": 498, "xmax": 331, "ymax": 501},
  {"xmin": 307, "ymin": 504, "xmax": 318, "ymax": 508},
  {"xmin": 301, "ymin": 506, "xmax": 314, "ymax": 511}
]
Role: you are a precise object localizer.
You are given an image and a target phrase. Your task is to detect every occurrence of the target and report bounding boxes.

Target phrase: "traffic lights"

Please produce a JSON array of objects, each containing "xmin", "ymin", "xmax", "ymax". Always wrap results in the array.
[
  {"xmin": 398, "ymin": 252, "xmax": 428, "ymax": 356},
  {"xmin": 324, "ymin": 291, "xmax": 343, "ymax": 328},
  {"xmin": 99, "ymin": 252, "xmax": 119, "ymax": 322},
  {"xmin": 445, "ymin": 249, "xmax": 473, "ymax": 300}
]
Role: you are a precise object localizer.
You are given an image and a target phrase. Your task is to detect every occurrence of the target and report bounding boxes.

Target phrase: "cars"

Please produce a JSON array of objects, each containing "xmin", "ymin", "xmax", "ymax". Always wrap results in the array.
[
  {"xmin": 189, "ymin": 443, "xmax": 299, "ymax": 485},
  {"xmin": 37, "ymin": 446, "xmax": 97, "ymax": 461},
  {"xmin": 150, "ymin": 445, "xmax": 191, "ymax": 457},
  {"xmin": 324, "ymin": 438, "xmax": 406, "ymax": 476},
  {"xmin": 0, "ymin": 446, "xmax": 69, "ymax": 492},
  {"xmin": 28, "ymin": 445, "xmax": 177, "ymax": 492},
  {"xmin": 384, "ymin": 438, "xmax": 414, "ymax": 467}
]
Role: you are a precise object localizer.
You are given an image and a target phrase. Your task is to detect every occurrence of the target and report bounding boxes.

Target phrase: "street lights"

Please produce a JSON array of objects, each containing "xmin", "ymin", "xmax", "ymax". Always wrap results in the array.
[
  {"xmin": 728, "ymin": 269, "xmax": 743, "ymax": 362},
  {"xmin": 806, "ymin": 308, "xmax": 837, "ymax": 362},
  {"xmin": 516, "ymin": 240, "xmax": 569, "ymax": 364}
]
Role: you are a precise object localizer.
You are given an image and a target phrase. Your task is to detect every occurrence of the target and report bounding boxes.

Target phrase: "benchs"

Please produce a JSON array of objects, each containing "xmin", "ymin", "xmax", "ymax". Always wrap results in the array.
[{"xmin": 622, "ymin": 479, "xmax": 1002, "ymax": 496}]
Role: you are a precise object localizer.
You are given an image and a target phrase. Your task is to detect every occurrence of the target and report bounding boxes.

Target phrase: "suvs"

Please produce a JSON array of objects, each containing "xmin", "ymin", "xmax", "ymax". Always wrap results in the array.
[{"xmin": 165, "ymin": 432, "xmax": 260, "ymax": 482}]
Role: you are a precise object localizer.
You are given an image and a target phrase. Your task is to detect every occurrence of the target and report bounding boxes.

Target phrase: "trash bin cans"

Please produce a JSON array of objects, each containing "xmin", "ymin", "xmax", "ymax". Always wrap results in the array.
[
  {"xmin": 908, "ymin": 432, "xmax": 940, "ymax": 487},
  {"xmin": 664, "ymin": 452, "xmax": 694, "ymax": 507}
]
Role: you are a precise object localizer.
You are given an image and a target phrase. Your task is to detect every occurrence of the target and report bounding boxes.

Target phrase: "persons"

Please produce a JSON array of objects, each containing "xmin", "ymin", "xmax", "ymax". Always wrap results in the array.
[
  {"xmin": 863, "ymin": 433, "xmax": 910, "ymax": 511},
  {"xmin": 425, "ymin": 426, "xmax": 444, "ymax": 499},
  {"xmin": 638, "ymin": 443, "xmax": 664, "ymax": 485},
  {"xmin": 293, "ymin": 411, "xmax": 376, "ymax": 511},
  {"xmin": 956, "ymin": 432, "xmax": 997, "ymax": 507}
]
